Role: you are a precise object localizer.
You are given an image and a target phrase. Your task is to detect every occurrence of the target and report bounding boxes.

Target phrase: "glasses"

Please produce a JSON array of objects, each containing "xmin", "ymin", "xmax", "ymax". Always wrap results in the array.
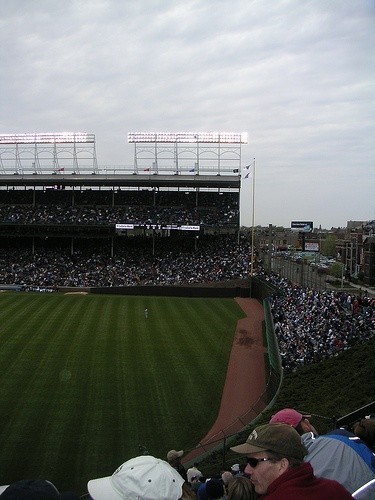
[{"xmin": 246, "ymin": 456, "xmax": 288, "ymax": 468}]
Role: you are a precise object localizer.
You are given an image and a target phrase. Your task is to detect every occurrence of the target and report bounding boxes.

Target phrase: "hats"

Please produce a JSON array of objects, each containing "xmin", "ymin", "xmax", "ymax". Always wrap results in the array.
[
  {"xmin": 167, "ymin": 450, "xmax": 183, "ymax": 460},
  {"xmin": 365, "ymin": 414, "xmax": 371, "ymax": 419},
  {"xmin": 229, "ymin": 423, "xmax": 305, "ymax": 463},
  {"xmin": 270, "ymin": 409, "xmax": 312, "ymax": 430},
  {"xmin": 231, "ymin": 463, "xmax": 240, "ymax": 472},
  {"xmin": 87, "ymin": 455, "xmax": 187, "ymax": 500},
  {"xmin": 0, "ymin": 479, "xmax": 60, "ymax": 500}
]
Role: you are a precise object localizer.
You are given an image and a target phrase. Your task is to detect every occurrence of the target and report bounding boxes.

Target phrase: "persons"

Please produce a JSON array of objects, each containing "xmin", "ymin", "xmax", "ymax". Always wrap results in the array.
[
  {"xmin": 145, "ymin": 307, "xmax": 148, "ymax": 319},
  {"xmin": 87, "ymin": 455, "xmax": 197, "ymax": 500},
  {"xmin": 187, "ymin": 462, "xmax": 202, "ymax": 483},
  {"xmin": 167, "ymin": 450, "xmax": 188, "ymax": 483},
  {"xmin": 0, "ymin": 189, "xmax": 238, "ymax": 227},
  {"xmin": 354, "ymin": 419, "xmax": 375, "ymax": 453},
  {"xmin": 191, "ymin": 463, "xmax": 259, "ymax": 500},
  {"xmin": 0, "ymin": 480, "xmax": 79, "ymax": 500},
  {"xmin": 230, "ymin": 423, "xmax": 355, "ymax": 500},
  {"xmin": 0, "ymin": 234, "xmax": 252, "ymax": 286},
  {"xmin": 21, "ymin": 284, "xmax": 59, "ymax": 293},
  {"xmin": 269, "ymin": 409, "xmax": 375, "ymax": 493},
  {"xmin": 251, "ymin": 246, "xmax": 375, "ymax": 372}
]
